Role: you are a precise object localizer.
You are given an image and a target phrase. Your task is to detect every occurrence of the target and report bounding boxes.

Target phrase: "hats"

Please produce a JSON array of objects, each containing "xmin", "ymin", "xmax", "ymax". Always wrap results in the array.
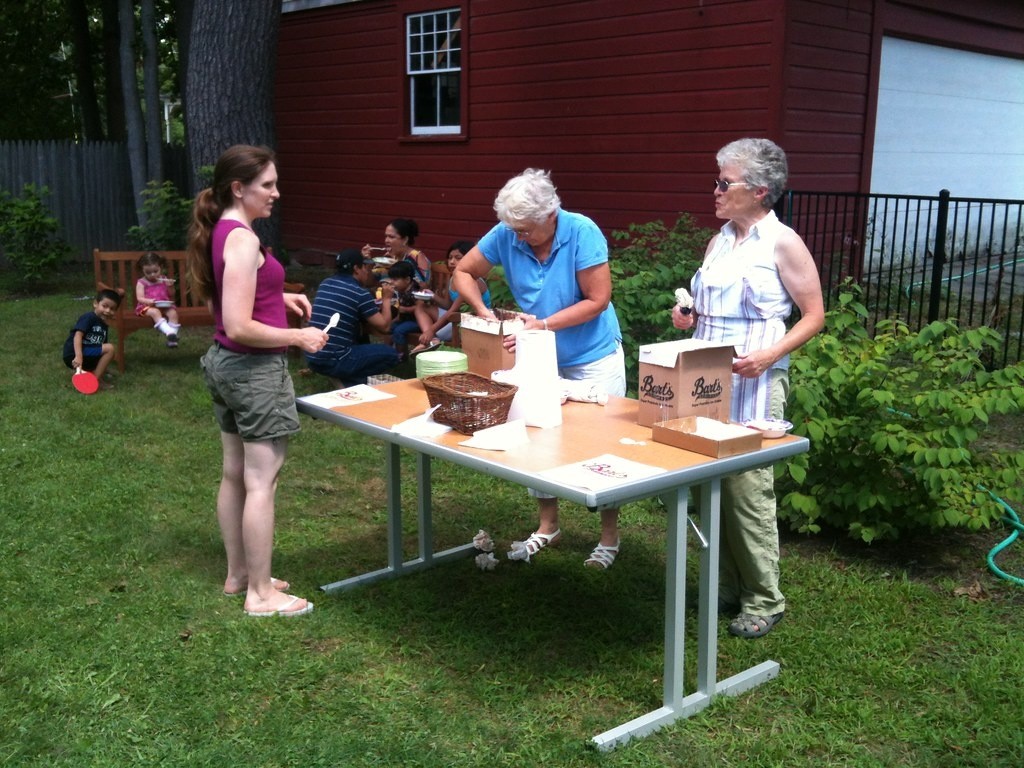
[{"xmin": 335, "ymin": 250, "xmax": 374, "ymax": 270}]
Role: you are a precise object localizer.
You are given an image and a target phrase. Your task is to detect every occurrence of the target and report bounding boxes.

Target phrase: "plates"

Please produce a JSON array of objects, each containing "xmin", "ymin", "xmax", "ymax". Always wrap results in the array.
[{"xmin": 371, "ymin": 257, "xmax": 397, "ymax": 265}]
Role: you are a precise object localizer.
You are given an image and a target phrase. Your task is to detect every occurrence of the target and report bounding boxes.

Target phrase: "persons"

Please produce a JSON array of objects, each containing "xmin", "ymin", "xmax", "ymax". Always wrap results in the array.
[
  {"xmin": 672, "ymin": 139, "xmax": 825, "ymax": 636},
  {"xmin": 184, "ymin": 144, "xmax": 329, "ymax": 617},
  {"xmin": 63, "ymin": 290, "xmax": 121, "ymax": 390},
  {"xmin": 135, "ymin": 252, "xmax": 182, "ymax": 348},
  {"xmin": 306, "ymin": 217, "xmax": 491, "ymax": 389},
  {"xmin": 453, "ymin": 169, "xmax": 627, "ymax": 571}
]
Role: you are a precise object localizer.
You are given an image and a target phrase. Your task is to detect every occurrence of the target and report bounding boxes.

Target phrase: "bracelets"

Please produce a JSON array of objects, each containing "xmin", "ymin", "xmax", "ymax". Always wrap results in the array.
[{"xmin": 544, "ymin": 319, "xmax": 548, "ymax": 331}]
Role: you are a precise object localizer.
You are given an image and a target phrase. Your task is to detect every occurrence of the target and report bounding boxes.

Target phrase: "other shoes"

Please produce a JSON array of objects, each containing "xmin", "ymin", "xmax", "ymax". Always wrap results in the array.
[{"xmin": 166, "ymin": 333, "xmax": 179, "ymax": 349}]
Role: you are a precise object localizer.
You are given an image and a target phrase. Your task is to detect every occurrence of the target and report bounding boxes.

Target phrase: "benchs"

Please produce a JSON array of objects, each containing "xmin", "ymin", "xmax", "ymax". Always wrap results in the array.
[
  {"xmin": 363, "ymin": 260, "xmax": 513, "ymax": 351},
  {"xmin": 91, "ymin": 248, "xmax": 305, "ymax": 371}
]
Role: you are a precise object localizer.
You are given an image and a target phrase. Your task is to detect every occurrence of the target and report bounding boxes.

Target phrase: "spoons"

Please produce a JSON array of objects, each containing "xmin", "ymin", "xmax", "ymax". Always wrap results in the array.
[{"xmin": 322, "ymin": 312, "xmax": 341, "ymax": 334}]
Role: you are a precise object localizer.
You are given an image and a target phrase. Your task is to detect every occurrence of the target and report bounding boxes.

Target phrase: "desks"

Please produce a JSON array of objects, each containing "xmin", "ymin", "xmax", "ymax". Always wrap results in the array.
[{"xmin": 287, "ymin": 374, "xmax": 812, "ymax": 754}]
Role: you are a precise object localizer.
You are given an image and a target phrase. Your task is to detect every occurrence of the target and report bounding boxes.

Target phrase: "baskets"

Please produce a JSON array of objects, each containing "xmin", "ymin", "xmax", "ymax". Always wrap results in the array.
[{"xmin": 421, "ymin": 371, "xmax": 518, "ymax": 435}]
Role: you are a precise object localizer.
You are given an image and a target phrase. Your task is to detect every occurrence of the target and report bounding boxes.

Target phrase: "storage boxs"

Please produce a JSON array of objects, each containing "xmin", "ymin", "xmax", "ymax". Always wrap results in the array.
[
  {"xmin": 636, "ymin": 338, "xmax": 741, "ymax": 426},
  {"xmin": 652, "ymin": 412, "xmax": 765, "ymax": 456},
  {"xmin": 460, "ymin": 308, "xmax": 544, "ymax": 378}
]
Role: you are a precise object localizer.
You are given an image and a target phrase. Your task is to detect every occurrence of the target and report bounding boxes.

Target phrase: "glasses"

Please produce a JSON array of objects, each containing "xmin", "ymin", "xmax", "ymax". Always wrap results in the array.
[
  {"xmin": 506, "ymin": 223, "xmax": 539, "ymax": 239},
  {"xmin": 714, "ymin": 180, "xmax": 751, "ymax": 193}
]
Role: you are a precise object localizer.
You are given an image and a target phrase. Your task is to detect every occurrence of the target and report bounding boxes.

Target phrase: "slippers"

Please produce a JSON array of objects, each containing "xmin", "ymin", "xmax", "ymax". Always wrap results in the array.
[
  {"xmin": 243, "ymin": 594, "xmax": 315, "ymax": 617},
  {"xmin": 242, "ymin": 594, "xmax": 314, "ymax": 617},
  {"xmin": 222, "ymin": 578, "xmax": 290, "ymax": 597}
]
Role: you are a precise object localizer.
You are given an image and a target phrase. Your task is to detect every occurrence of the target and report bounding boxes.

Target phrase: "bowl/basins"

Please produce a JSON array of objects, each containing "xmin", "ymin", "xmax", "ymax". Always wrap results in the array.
[
  {"xmin": 154, "ymin": 301, "xmax": 174, "ymax": 308},
  {"xmin": 416, "ymin": 350, "xmax": 468, "ymax": 381},
  {"xmin": 742, "ymin": 418, "xmax": 794, "ymax": 438},
  {"xmin": 412, "ymin": 291, "xmax": 434, "ymax": 300}
]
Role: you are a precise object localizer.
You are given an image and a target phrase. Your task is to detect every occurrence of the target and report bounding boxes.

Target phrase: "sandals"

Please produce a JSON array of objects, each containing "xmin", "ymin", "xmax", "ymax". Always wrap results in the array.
[
  {"xmin": 525, "ymin": 529, "xmax": 560, "ymax": 554},
  {"xmin": 692, "ymin": 595, "xmax": 732, "ymax": 613},
  {"xmin": 728, "ymin": 612, "xmax": 783, "ymax": 638},
  {"xmin": 409, "ymin": 337, "xmax": 441, "ymax": 360},
  {"xmin": 583, "ymin": 539, "xmax": 621, "ymax": 571}
]
